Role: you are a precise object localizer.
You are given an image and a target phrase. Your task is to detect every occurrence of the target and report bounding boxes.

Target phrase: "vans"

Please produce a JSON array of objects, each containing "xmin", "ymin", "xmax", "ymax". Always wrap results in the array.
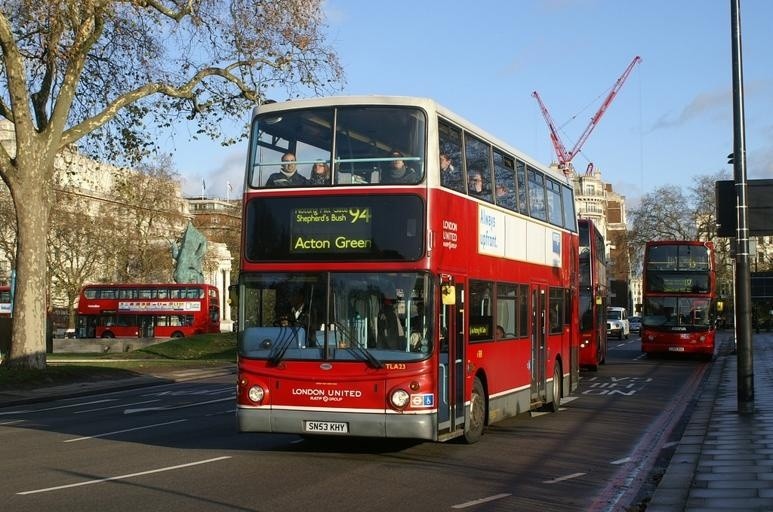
[{"xmin": 605, "ymin": 306, "xmax": 633, "ymax": 339}]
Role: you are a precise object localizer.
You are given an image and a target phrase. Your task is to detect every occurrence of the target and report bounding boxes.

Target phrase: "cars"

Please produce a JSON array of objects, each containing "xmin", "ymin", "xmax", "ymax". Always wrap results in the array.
[{"xmin": 627, "ymin": 315, "xmax": 643, "ymax": 333}]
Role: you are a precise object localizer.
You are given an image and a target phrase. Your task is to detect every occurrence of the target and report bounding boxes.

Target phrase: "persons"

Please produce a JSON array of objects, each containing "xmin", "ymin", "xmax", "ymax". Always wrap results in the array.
[
  {"xmin": 382, "ymin": 148, "xmax": 517, "ymax": 209},
  {"xmin": 272, "ymin": 288, "xmax": 312, "ymax": 340},
  {"xmin": 265, "ymin": 153, "xmax": 331, "ymax": 188},
  {"xmin": 496, "ymin": 326, "xmax": 505, "ymax": 338},
  {"xmin": 409, "ymin": 301, "xmax": 424, "ymax": 351}
]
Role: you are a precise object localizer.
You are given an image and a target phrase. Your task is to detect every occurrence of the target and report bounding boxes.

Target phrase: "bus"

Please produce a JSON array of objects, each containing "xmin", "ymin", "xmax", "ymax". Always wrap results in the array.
[
  {"xmin": 74, "ymin": 282, "xmax": 220, "ymax": 340},
  {"xmin": 641, "ymin": 237, "xmax": 722, "ymax": 364}
]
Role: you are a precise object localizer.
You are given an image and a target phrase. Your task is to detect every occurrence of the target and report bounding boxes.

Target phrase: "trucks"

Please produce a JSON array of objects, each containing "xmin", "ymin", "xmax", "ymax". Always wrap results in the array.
[{"xmin": 0, "ymin": 285, "xmax": 70, "ymax": 339}]
